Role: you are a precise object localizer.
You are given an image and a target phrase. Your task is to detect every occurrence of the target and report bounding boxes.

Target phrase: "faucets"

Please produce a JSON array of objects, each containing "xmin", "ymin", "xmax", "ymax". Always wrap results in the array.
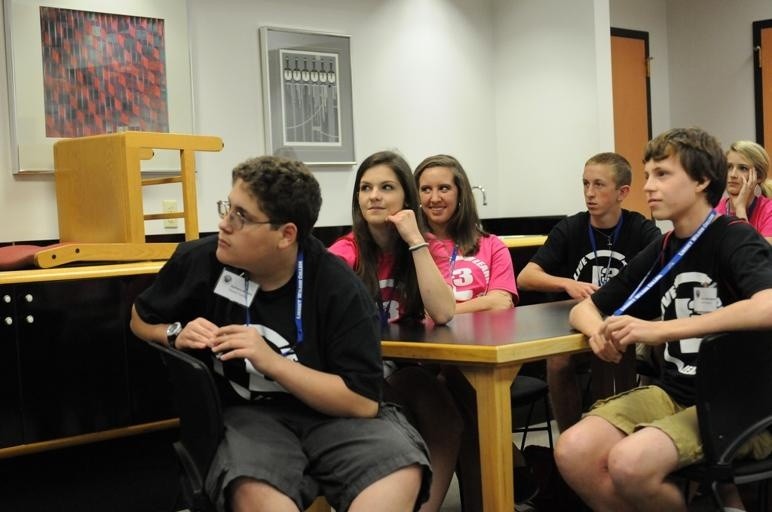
[{"xmin": 470, "ymin": 186, "xmax": 489, "ymax": 207}]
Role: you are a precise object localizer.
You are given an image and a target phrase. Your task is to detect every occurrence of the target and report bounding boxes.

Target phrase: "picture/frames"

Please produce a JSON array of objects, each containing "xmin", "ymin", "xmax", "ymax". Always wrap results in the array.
[
  {"xmin": 259, "ymin": 24, "xmax": 357, "ymax": 168},
  {"xmin": 4, "ymin": 0, "xmax": 198, "ymax": 177}
]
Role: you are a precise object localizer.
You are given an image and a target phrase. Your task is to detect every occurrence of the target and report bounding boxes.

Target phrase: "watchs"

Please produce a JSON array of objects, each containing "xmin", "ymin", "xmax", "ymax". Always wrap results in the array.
[{"xmin": 166, "ymin": 321, "xmax": 183, "ymax": 351}]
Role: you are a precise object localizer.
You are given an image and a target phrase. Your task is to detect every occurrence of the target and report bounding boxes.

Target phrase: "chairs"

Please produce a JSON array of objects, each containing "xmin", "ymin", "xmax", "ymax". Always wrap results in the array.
[
  {"xmin": 140, "ymin": 293, "xmax": 772, "ymax": 512},
  {"xmin": 0, "ymin": 129, "xmax": 223, "ymax": 269}
]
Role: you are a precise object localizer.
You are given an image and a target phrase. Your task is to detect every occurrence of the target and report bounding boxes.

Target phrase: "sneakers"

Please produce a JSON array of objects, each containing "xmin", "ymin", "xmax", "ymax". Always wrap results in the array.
[{"xmin": 513, "ymin": 450, "xmax": 538, "ymax": 506}]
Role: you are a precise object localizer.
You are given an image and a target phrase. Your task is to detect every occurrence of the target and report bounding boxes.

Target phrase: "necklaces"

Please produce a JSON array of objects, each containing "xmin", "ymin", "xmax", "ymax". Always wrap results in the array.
[{"xmin": 592, "ymin": 226, "xmax": 617, "ymax": 249}]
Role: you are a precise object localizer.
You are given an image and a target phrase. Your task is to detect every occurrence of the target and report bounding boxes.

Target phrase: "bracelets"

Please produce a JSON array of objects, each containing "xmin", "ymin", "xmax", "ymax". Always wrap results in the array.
[{"xmin": 408, "ymin": 242, "xmax": 429, "ymax": 251}]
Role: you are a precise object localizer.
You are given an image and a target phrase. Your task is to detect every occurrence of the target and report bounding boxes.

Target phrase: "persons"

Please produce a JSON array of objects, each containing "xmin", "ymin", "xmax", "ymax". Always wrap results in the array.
[
  {"xmin": 552, "ymin": 125, "xmax": 772, "ymax": 512},
  {"xmin": 712, "ymin": 140, "xmax": 772, "ymax": 247},
  {"xmin": 516, "ymin": 152, "xmax": 663, "ymax": 436},
  {"xmin": 324, "ymin": 150, "xmax": 466, "ymax": 512},
  {"xmin": 409, "ymin": 153, "xmax": 541, "ymax": 512},
  {"xmin": 128, "ymin": 153, "xmax": 434, "ymax": 512}
]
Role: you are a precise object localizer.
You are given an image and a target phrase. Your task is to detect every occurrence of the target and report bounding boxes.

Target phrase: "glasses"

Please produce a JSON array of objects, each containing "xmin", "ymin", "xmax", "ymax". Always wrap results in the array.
[{"xmin": 217, "ymin": 200, "xmax": 287, "ymax": 230}]
